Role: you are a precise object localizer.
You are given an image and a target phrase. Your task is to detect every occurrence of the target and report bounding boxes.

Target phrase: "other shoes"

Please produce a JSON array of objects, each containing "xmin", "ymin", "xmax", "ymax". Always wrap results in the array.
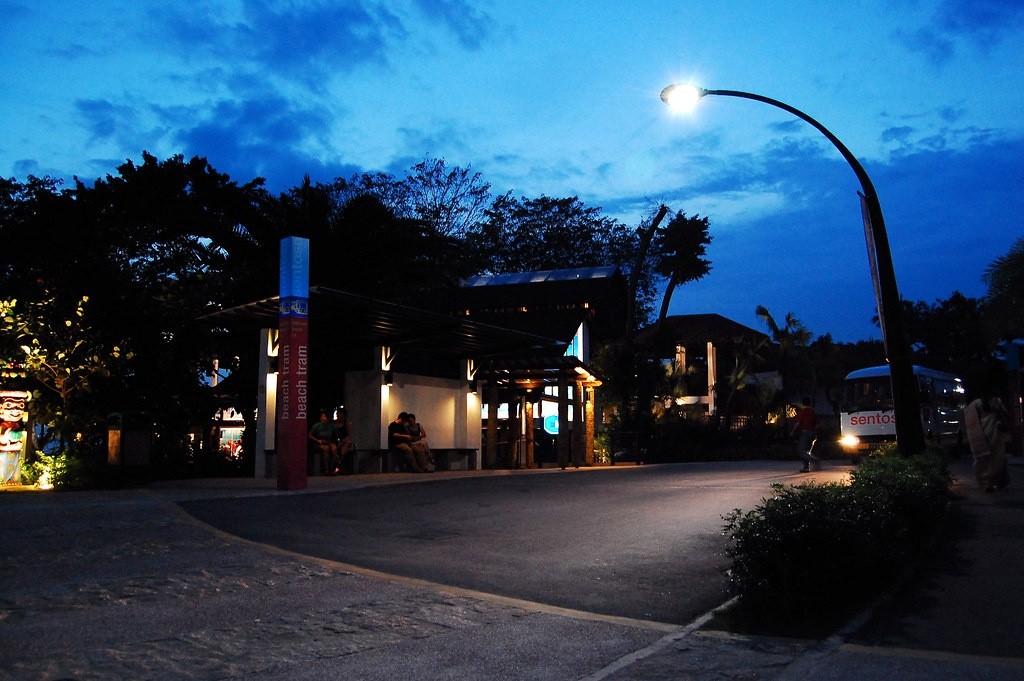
[
  {"xmin": 413, "ymin": 467, "xmax": 421, "ymax": 473},
  {"xmin": 799, "ymin": 468, "xmax": 809, "ymax": 473},
  {"xmin": 985, "ymin": 486, "xmax": 993, "ymax": 492},
  {"xmin": 998, "ymin": 481, "xmax": 1009, "ymax": 489},
  {"xmin": 423, "ymin": 468, "xmax": 434, "ymax": 473}
]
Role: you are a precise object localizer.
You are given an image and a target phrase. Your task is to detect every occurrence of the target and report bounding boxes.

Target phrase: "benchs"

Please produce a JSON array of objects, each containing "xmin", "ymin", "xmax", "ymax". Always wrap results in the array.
[{"xmin": 311, "ymin": 446, "xmax": 480, "ymax": 476}]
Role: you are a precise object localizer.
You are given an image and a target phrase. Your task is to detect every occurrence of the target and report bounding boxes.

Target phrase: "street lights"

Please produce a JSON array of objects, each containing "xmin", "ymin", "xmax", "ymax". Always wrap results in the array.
[{"xmin": 657, "ymin": 82, "xmax": 924, "ymax": 459}]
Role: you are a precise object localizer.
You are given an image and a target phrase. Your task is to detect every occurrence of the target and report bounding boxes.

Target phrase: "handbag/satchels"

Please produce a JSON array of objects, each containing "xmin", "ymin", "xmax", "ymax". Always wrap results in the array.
[{"xmin": 992, "ymin": 396, "xmax": 1009, "ymax": 431}]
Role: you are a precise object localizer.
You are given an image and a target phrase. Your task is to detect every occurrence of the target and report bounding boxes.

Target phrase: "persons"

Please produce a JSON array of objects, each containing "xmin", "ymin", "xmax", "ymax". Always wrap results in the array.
[
  {"xmin": 308, "ymin": 409, "xmax": 354, "ymax": 475},
  {"xmin": 791, "ymin": 398, "xmax": 821, "ymax": 473},
  {"xmin": 388, "ymin": 412, "xmax": 439, "ymax": 472}
]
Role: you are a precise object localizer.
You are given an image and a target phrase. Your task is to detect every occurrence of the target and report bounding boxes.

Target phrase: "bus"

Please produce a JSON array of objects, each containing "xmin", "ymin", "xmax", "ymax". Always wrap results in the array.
[{"xmin": 838, "ymin": 363, "xmax": 964, "ymax": 465}]
